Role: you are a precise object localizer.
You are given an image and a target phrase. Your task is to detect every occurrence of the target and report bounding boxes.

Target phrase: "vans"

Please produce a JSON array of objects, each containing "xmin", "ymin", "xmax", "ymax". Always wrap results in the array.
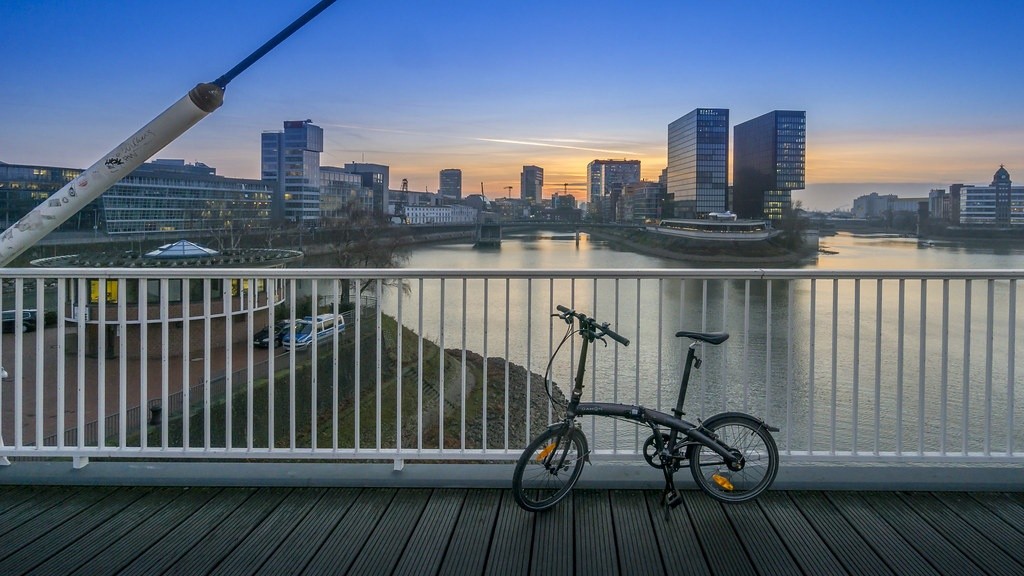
[{"xmin": 283, "ymin": 314, "xmax": 345, "ymax": 351}]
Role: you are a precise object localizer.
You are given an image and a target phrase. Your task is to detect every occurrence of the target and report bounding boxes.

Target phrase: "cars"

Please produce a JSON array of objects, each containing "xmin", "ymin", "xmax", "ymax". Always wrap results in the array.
[
  {"xmin": 254, "ymin": 318, "xmax": 303, "ymax": 349},
  {"xmin": 1, "ymin": 309, "xmax": 37, "ymax": 334}
]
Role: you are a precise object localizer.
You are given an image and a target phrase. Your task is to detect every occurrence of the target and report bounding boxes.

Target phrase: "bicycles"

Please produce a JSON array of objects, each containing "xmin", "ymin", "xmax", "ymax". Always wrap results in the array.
[{"xmin": 512, "ymin": 306, "xmax": 780, "ymax": 512}]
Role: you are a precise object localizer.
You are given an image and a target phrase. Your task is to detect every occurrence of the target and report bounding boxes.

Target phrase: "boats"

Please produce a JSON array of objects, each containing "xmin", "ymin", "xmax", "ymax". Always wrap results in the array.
[{"xmin": 474, "ymin": 182, "xmax": 502, "ymax": 250}]
